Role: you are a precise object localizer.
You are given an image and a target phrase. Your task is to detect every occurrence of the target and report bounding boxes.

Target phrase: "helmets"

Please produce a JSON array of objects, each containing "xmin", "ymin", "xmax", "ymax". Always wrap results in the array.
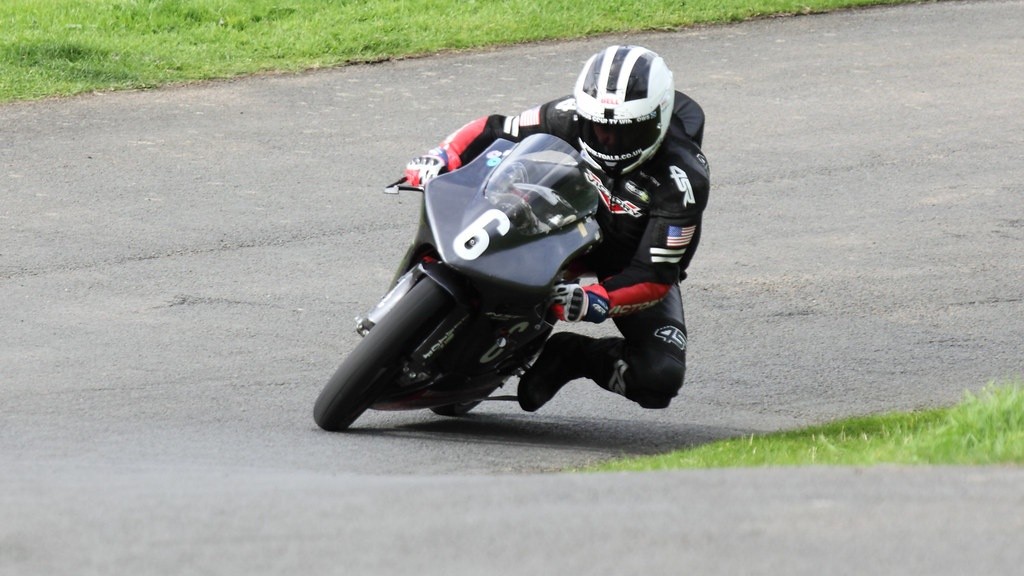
[{"xmin": 573, "ymin": 45, "xmax": 675, "ymax": 177}]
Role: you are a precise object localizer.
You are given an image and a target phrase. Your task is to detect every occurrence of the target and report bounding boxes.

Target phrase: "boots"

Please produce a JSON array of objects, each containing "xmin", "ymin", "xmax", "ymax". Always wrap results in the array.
[{"xmin": 517, "ymin": 331, "xmax": 635, "ymax": 413}]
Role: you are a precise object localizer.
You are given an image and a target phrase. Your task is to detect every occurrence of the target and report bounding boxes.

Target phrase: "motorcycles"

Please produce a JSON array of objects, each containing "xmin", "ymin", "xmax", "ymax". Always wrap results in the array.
[{"xmin": 314, "ymin": 90, "xmax": 705, "ymax": 432}]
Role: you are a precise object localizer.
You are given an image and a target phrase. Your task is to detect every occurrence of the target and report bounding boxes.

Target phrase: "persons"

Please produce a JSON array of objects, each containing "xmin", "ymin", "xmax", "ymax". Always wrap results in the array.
[{"xmin": 402, "ymin": 43, "xmax": 712, "ymax": 412}]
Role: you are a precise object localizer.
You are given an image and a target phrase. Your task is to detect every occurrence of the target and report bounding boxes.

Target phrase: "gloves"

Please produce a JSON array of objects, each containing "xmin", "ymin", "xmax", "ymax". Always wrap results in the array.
[
  {"xmin": 407, "ymin": 155, "xmax": 444, "ymax": 187},
  {"xmin": 548, "ymin": 283, "xmax": 589, "ymax": 322}
]
